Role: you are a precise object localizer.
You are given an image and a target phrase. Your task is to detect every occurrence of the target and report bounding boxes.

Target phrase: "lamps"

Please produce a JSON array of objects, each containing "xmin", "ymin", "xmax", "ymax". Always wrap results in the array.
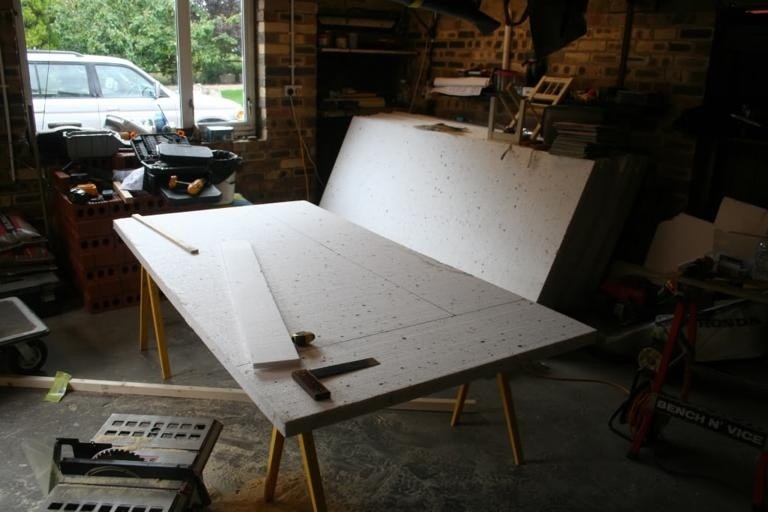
[
  {"xmin": 401, "ymin": 1, "xmax": 501, "ymax": 35},
  {"xmin": 318, "ymin": 7, "xmax": 403, "ymax": 29}
]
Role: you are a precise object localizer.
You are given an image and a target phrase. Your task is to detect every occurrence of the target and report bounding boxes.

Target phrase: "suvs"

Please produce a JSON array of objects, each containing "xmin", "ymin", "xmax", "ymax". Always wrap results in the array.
[{"xmin": 27, "ymin": 50, "xmax": 244, "ymax": 137}]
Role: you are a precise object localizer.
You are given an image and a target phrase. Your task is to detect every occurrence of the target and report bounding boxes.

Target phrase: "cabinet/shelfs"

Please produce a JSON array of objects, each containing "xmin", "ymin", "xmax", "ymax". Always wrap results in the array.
[{"xmin": 317, "ymin": 47, "xmax": 420, "ymax": 120}]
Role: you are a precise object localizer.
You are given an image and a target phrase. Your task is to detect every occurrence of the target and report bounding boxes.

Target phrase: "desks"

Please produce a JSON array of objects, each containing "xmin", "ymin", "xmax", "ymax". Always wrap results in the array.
[{"xmin": 112, "ymin": 198, "xmax": 599, "ymax": 512}]
